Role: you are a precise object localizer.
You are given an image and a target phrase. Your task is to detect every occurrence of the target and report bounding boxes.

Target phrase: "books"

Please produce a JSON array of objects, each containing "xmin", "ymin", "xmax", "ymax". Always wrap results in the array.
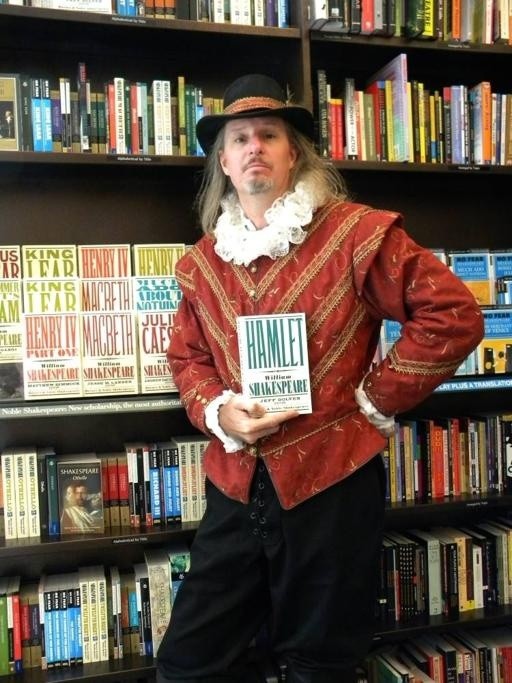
[
  {"xmin": 366, "ymin": 622, "xmax": 512, "ymax": 683},
  {"xmin": 236, "ymin": 313, "xmax": 314, "ymax": 414},
  {"xmin": 382, "ymin": 250, "xmax": 512, "ymax": 375},
  {"xmin": 1, "ymin": 243, "xmax": 195, "ymax": 399},
  {"xmin": 316, "ymin": 54, "xmax": 512, "ymax": 166},
  {"xmin": 381, "ymin": 413, "xmax": 512, "ymax": 502},
  {"xmin": 0, "ymin": 548, "xmax": 190, "ymax": 676},
  {"xmin": 380, "ymin": 515, "xmax": 512, "ymax": 621},
  {"xmin": 7, "ymin": 0, "xmax": 289, "ymax": 29},
  {"xmin": 308, "ymin": 0, "xmax": 512, "ymax": 47},
  {"xmin": 0, "ymin": 64, "xmax": 223, "ymax": 158},
  {"xmin": 0, "ymin": 436, "xmax": 211, "ymax": 539}
]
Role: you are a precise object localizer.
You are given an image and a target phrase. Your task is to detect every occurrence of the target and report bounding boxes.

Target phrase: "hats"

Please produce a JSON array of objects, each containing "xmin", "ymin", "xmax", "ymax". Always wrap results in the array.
[{"xmin": 195, "ymin": 74, "xmax": 313, "ymax": 158}]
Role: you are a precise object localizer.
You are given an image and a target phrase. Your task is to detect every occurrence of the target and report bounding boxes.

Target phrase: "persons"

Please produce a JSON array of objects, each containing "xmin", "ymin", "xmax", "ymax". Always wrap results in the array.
[{"xmin": 157, "ymin": 75, "xmax": 485, "ymax": 683}]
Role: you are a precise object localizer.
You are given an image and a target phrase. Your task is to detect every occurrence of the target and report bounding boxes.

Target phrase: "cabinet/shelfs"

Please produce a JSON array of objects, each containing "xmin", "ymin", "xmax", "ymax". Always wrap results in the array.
[{"xmin": 0, "ymin": 0, "xmax": 512, "ymax": 683}]
